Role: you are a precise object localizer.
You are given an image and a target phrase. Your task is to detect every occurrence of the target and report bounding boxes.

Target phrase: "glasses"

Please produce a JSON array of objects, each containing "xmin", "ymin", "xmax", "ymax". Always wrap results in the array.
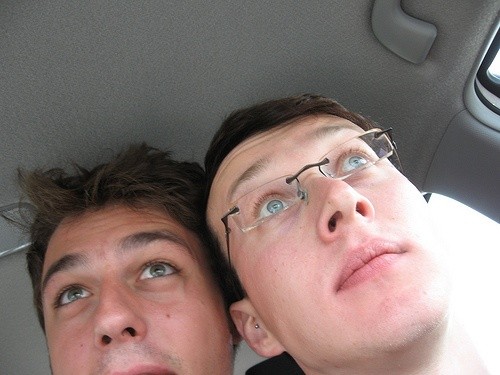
[{"xmin": 220, "ymin": 126, "xmax": 405, "ymax": 301}]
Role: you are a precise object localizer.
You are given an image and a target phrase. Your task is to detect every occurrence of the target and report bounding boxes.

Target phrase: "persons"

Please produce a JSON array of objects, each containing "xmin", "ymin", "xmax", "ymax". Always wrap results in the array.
[
  {"xmin": 0, "ymin": 142, "xmax": 247, "ymax": 375},
  {"xmin": 198, "ymin": 93, "xmax": 492, "ymax": 375}
]
